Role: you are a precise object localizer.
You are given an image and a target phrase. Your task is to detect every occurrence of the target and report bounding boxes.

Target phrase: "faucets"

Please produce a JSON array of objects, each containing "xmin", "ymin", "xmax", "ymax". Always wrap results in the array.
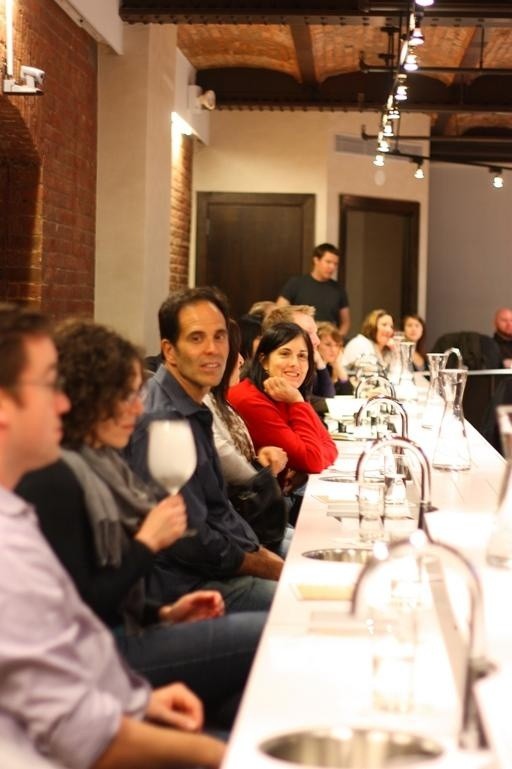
[
  {"xmin": 354, "ymin": 376, "xmax": 394, "ymax": 416},
  {"xmin": 354, "ymin": 436, "xmax": 438, "ymax": 531},
  {"xmin": 356, "ymin": 396, "xmax": 410, "ymax": 487},
  {"xmin": 351, "ymin": 353, "xmax": 387, "ymax": 378},
  {"xmin": 347, "ymin": 539, "xmax": 497, "ymax": 751}
]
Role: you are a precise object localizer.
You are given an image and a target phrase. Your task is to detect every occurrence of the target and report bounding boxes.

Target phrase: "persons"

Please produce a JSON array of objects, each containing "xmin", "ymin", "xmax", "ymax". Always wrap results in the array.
[
  {"xmin": 122, "ymin": 285, "xmax": 285, "ymax": 613},
  {"xmin": 492, "ymin": 307, "xmax": 512, "ymax": 403},
  {"xmin": 16, "ymin": 317, "xmax": 270, "ymax": 733},
  {"xmin": 204, "ymin": 244, "xmax": 434, "ymax": 555},
  {"xmin": 2, "ymin": 298, "xmax": 226, "ymax": 769}
]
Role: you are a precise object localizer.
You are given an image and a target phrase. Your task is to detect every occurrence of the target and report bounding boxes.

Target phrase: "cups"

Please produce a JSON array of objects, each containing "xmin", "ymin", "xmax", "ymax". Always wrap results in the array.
[{"xmin": 357, "ymin": 482, "xmax": 386, "ymax": 545}]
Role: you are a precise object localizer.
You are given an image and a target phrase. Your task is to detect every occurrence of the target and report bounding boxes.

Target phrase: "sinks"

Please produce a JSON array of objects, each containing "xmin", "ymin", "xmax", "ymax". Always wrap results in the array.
[
  {"xmin": 319, "ymin": 474, "xmax": 384, "ymax": 484},
  {"xmin": 302, "ymin": 545, "xmax": 375, "ymax": 563},
  {"xmin": 259, "ymin": 725, "xmax": 446, "ymax": 767}
]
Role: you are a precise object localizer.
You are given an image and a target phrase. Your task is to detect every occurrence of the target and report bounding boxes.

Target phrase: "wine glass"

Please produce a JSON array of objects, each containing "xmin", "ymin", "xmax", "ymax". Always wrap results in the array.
[{"xmin": 144, "ymin": 417, "xmax": 198, "ymax": 539}]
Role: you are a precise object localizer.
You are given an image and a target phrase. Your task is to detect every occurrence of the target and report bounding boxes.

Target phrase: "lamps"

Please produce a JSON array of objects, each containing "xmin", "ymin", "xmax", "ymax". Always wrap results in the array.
[
  {"xmin": 395, "ymin": 78, "xmax": 408, "ymax": 101},
  {"xmin": 403, "ymin": 47, "xmax": 418, "ymax": 71},
  {"xmin": 489, "ymin": 167, "xmax": 503, "ymax": 189},
  {"xmin": 387, "ymin": 100, "xmax": 400, "ymax": 119},
  {"xmin": 374, "ymin": 151, "xmax": 384, "ymax": 167},
  {"xmin": 408, "ymin": 15, "xmax": 425, "ymax": 46},
  {"xmin": 377, "ymin": 137, "xmax": 389, "ymax": 153},
  {"xmin": 409, "ymin": 157, "xmax": 424, "ymax": 179},
  {"xmin": 382, "ymin": 119, "xmax": 394, "ymax": 137}
]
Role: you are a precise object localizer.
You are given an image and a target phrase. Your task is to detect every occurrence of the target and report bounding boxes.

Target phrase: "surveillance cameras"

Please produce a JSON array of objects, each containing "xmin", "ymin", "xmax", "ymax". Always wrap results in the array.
[{"xmin": 2, "ymin": 64, "xmax": 46, "ymax": 102}]
